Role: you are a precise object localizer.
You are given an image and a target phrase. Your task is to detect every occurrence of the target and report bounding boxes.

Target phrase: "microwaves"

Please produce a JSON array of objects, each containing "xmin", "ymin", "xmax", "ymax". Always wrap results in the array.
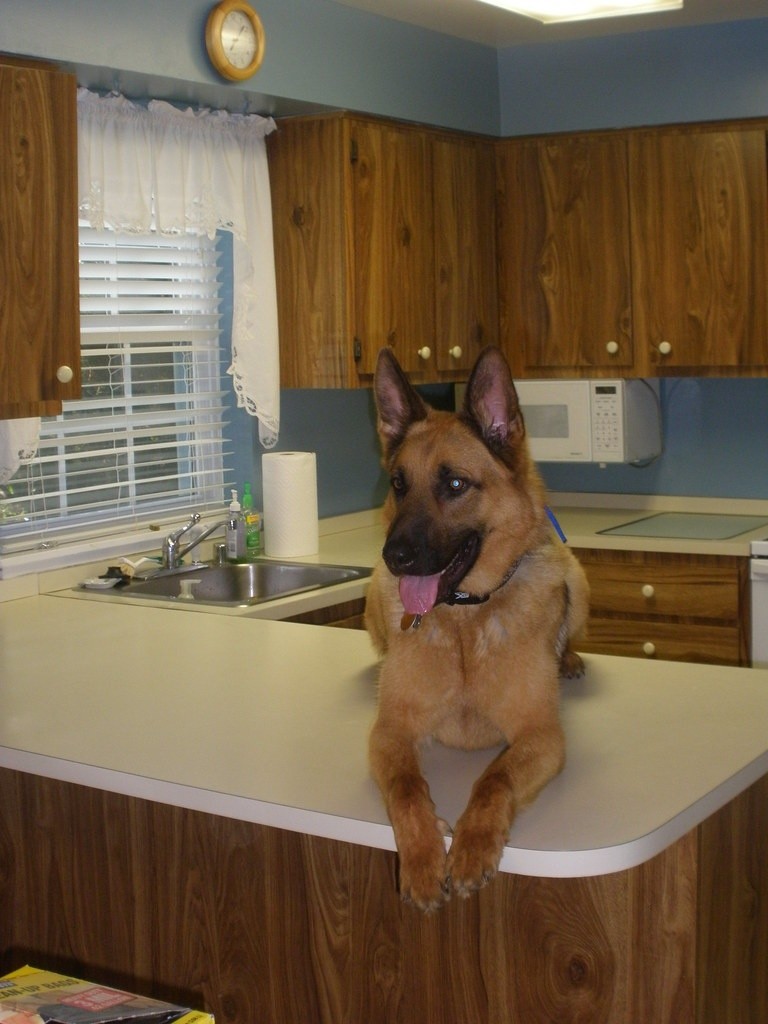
[{"xmin": 456, "ymin": 378, "xmax": 662, "ymax": 467}]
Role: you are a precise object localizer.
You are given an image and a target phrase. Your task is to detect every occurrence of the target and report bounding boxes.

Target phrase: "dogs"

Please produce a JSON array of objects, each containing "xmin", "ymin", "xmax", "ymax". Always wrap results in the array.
[{"xmin": 363, "ymin": 342, "xmax": 592, "ymax": 914}]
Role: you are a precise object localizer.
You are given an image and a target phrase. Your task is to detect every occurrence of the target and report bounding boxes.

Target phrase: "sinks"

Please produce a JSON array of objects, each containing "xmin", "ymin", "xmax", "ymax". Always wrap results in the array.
[{"xmin": 119, "ymin": 561, "xmax": 375, "ymax": 608}]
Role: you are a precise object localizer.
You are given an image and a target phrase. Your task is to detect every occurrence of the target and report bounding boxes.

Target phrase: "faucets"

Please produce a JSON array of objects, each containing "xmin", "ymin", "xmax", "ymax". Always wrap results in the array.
[{"xmin": 161, "ymin": 513, "xmax": 238, "ymax": 571}]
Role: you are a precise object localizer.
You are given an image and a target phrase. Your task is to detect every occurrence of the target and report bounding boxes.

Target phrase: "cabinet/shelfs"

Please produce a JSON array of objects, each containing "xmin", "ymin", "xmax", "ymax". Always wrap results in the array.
[
  {"xmin": 0, "ymin": 52, "xmax": 84, "ymax": 422},
  {"xmin": 280, "ymin": 548, "xmax": 753, "ymax": 670},
  {"xmin": 267, "ymin": 110, "xmax": 768, "ymax": 390}
]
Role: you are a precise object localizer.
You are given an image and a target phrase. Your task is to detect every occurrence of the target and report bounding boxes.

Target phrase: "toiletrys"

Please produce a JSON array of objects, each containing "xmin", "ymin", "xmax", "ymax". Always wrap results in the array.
[{"xmin": 225, "ymin": 483, "xmax": 261, "ymax": 561}]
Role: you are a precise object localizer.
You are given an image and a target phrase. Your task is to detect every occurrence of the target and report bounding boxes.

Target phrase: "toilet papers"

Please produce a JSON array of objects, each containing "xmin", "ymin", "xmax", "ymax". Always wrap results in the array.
[{"xmin": 261, "ymin": 451, "xmax": 319, "ymax": 558}]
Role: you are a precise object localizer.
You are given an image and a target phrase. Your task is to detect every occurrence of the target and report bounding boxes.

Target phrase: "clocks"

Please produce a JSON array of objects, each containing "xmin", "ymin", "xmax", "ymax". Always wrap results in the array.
[{"xmin": 206, "ymin": 0, "xmax": 266, "ymax": 83}]
